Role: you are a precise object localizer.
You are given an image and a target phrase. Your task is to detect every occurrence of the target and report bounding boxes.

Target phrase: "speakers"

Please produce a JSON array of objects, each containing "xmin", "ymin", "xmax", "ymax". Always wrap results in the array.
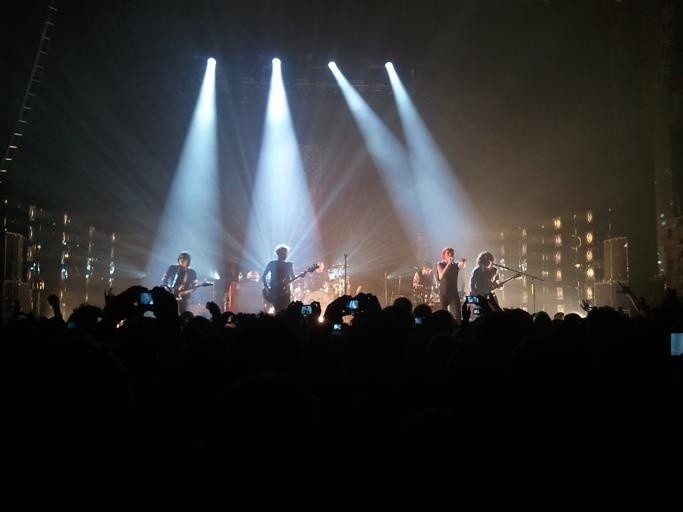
[
  {"xmin": 0, "ymin": 229, "xmax": 37, "ymax": 315},
  {"xmin": 591, "ymin": 236, "xmax": 639, "ymax": 315}
]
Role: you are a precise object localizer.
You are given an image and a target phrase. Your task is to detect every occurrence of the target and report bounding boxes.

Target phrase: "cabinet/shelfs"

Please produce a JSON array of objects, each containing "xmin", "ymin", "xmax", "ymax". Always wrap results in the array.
[{"xmin": 591, "ymin": 235, "xmax": 632, "ymax": 313}]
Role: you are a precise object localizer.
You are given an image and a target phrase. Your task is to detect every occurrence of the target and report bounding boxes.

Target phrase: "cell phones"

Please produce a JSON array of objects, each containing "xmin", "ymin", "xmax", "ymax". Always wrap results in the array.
[
  {"xmin": 301, "ymin": 305, "xmax": 312, "ymax": 316},
  {"xmin": 466, "ymin": 296, "xmax": 480, "ymax": 304},
  {"xmin": 414, "ymin": 316, "xmax": 423, "ymax": 326},
  {"xmin": 667, "ymin": 330, "xmax": 683, "ymax": 359},
  {"xmin": 138, "ymin": 292, "xmax": 155, "ymax": 307},
  {"xmin": 333, "ymin": 324, "xmax": 340, "ymax": 332},
  {"xmin": 472, "ymin": 308, "xmax": 481, "ymax": 315},
  {"xmin": 344, "ymin": 299, "xmax": 360, "ymax": 311}
]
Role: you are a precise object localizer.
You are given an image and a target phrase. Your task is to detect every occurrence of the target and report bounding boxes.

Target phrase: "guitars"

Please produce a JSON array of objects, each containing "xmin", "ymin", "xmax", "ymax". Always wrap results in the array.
[
  {"xmin": 164, "ymin": 279, "xmax": 213, "ymax": 301},
  {"xmin": 470, "ymin": 271, "xmax": 522, "ymax": 304},
  {"xmin": 262, "ymin": 265, "xmax": 319, "ymax": 303}
]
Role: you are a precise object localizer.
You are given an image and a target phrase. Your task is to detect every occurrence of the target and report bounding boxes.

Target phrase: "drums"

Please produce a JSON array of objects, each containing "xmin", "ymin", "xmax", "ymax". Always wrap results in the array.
[
  {"xmin": 302, "ymin": 291, "xmax": 334, "ymax": 322},
  {"xmin": 413, "ymin": 286, "xmax": 433, "ymax": 307}
]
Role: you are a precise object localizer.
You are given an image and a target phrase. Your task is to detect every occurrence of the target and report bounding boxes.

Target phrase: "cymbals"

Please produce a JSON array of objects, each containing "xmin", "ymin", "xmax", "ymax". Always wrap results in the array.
[{"xmin": 331, "ymin": 264, "xmax": 352, "ymax": 268}]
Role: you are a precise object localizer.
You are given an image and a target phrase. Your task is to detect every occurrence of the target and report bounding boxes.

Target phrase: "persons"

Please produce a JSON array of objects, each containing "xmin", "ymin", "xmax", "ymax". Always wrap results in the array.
[
  {"xmin": 159, "ymin": 252, "xmax": 197, "ymax": 313},
  {"xmin": 470, "ymin": 251, "xmax": 504, "ymax": 312},
  {"xmin": 412, "ymin": 264, "xmax": 439, "ymax": 292},
  {"xmin": 262, "ymin": 246, "xmax": 305, "ymax": 314},
  {"xmin": 1, "ymin": 281, "xmax": 683, "ymax": 511},
  {"xmin": 436, "ymin": 248, "xmax": 466, "ymax": 323}
]
